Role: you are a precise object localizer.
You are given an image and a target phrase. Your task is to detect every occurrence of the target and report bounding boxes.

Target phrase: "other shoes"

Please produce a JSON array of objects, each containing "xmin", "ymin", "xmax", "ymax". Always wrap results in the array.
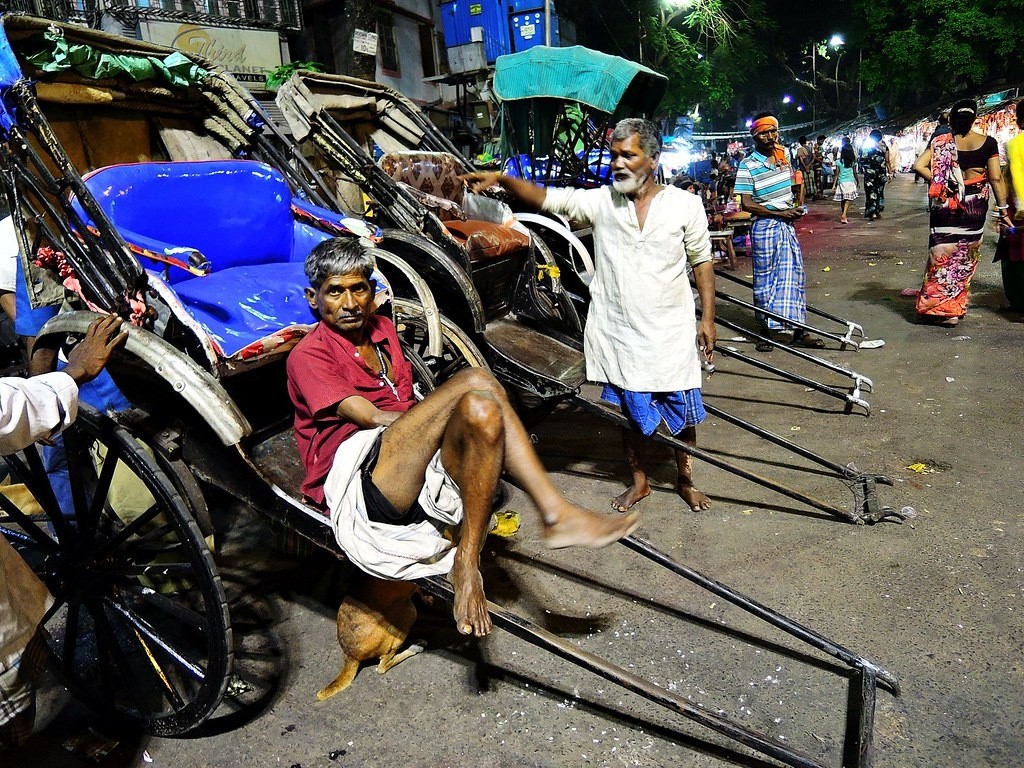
[{"xmin": 934, "ymin": 315, "xmax": 960, "ymax": 324}]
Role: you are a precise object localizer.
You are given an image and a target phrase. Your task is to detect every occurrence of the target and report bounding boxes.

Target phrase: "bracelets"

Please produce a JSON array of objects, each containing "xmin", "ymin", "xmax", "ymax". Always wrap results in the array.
[
  {"xmin": 1001, "ymin": 215, "xmax": 1009, "ymax": 217},
  {"xmin": 995, "ymin": 203, "xmax": 1009, "ymax": 210}
]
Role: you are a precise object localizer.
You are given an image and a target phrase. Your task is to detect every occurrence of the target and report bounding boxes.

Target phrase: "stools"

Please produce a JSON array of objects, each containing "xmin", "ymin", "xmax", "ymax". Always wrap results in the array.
[{"xmin": 710, "ymin": 231, "xmax": 736, "ymax": 271}]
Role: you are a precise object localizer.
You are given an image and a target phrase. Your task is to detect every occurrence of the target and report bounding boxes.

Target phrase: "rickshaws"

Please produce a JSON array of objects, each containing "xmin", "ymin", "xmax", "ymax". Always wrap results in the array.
[
  {"xmin": 490, "ymin": 43, "xmax": 888, "ymax": 352},
  {"xmin": 0, "ymin": 9, "xmax": 901, "ymax": 768},
  {"xmin": 272, "ymin": 67, "xmax": 918, "ymax": 529}
]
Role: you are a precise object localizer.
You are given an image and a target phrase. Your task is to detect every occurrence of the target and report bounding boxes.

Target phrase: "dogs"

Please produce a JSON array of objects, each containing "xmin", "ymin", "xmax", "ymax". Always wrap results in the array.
[{"xmin": 317, "ymin": 565, "xmax": 427, "ymax": 701}]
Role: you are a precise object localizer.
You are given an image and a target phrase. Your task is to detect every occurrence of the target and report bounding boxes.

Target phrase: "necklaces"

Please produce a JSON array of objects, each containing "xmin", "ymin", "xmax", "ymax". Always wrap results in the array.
[{"xmin": 366, "ymin": 338, "xmax": 399, "ymax": 402}]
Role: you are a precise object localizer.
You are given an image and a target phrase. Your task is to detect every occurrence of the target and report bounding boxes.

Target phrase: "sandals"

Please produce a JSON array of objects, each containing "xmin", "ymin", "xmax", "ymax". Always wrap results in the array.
[
  {"xmin": 793, "ymin": 334, "xmax": 825, "ymax": 349},
  {"xmin": 756, "ymin": 336, "xmax": 774, "ymax": 352}
]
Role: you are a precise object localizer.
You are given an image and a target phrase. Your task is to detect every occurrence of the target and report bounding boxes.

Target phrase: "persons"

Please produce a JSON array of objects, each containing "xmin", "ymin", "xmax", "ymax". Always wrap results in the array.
[
  {"xmin": 0, "ymin": 200, "xmax": 132, "ymax": 533},
  {"xmin": 674, "ymin": 99, "xmax": 1024, "ymax": 350},
  {"xmin": 285, "ymin": 238, "xmax": 642, "ymax": 637},
  {"xmin": 461, "ymin": 118, "xmax": 717, "ymax": 512}
]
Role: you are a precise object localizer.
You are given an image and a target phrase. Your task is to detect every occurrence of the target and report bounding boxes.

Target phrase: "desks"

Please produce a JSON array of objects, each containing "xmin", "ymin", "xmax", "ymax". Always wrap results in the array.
[{"xmin": 722, "ymin": 218, "xmax": 752, "ymax": 255}]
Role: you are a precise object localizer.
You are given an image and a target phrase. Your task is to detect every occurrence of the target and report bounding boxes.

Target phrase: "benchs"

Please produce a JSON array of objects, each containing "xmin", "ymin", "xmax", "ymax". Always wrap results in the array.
[
  {"xmin": 503, "ymin": 153, "xmax": 601, "ymax": 239},
  {"xmin": 69, "ymin": 161, "xmax": 396, "ymax": 380},
  {"xmin": 381, "ymin": 151, "xmax": 532, "ymax": 266}
]
